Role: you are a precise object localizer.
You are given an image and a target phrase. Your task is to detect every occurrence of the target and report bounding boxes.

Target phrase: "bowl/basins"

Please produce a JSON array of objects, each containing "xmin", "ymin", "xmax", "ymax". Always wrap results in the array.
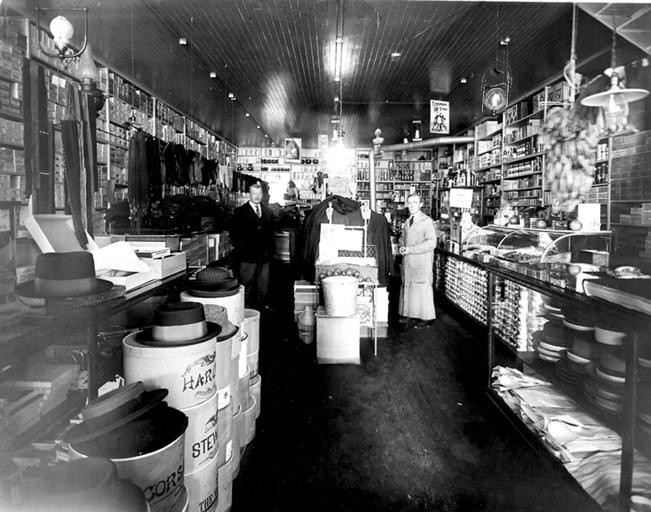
[{"xmin": 570, "ymin": 334, "xmax": 600, "ymax": 361}]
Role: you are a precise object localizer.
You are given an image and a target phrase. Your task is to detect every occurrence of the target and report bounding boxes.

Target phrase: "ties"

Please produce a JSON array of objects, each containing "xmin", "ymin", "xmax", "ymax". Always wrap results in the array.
[
  {"xmin": 255, "ymin": 205, "xmax": 261, "ymax": 218},
  {"xmin": 410, "ymin": 215, "xmax": 415, "ymax": 227}
]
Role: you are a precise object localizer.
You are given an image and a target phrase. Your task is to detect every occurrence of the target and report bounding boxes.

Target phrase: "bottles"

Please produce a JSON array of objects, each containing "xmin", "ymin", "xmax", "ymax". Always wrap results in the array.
[
  {"xmin": 488, "ymin": 119, "xmax": 544, "ymax": 179},
  {"xmin": 439, "ymin": 160, "xmax": 480, "ymax": 189},
  {"xmin": 357, "ymin": 154, "xmax": 414, "ymax": 207},
  {"xmin": 596, "ymin": 162, "xmax": 607, "ymax": 184}
]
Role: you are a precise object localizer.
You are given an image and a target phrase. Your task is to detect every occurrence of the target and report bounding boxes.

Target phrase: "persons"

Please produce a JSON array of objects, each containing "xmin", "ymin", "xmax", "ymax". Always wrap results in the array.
[
  {"xmin": 227, "ymin": 182, "xmax": 275, "ymax": 315},
  {"xmin": 395, "ymin": 193, "xmax": 437, "ymax": 330}
]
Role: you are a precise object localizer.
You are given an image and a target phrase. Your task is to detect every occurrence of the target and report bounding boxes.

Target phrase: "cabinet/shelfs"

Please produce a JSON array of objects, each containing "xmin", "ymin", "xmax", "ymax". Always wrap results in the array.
[
  {"xmin": 434, "ymin": 39, "xmax": 649, "ymax": 490},
  {"xmin": 2, "ymin": 1, "xmax": 239, "ymax": 512},
  {"xmin": 239, "ymin": 145, "xmax": 434, "ymax": 246}
]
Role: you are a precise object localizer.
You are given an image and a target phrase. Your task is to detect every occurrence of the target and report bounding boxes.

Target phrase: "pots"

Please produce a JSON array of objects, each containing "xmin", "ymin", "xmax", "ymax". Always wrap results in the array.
[{"xmin": 542, "ymin": 298, "xmax": 628, "ymax": 346}]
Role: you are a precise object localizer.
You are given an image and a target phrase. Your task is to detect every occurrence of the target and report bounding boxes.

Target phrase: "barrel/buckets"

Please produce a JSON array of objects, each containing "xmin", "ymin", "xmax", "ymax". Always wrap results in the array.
[{"xmin": 320, "ymin": 275, "xmax": 360, "ymax": 317}]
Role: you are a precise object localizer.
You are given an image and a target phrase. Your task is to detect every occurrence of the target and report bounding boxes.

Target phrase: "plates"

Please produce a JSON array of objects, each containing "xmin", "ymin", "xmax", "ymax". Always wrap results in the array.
[
  {"xmin": 535, "ymin": 341, "xmax": 625, "ymax": 414},
  {"xmin": 549, "ymin": 420, "xmax": 577, "ymax": 444}
]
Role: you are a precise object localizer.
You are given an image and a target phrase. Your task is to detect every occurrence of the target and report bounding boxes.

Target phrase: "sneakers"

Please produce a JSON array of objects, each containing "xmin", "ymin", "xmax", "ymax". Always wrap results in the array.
[{"xmin": 398, "ymin": 317, "xmax": 434, "ymax": 330}]
